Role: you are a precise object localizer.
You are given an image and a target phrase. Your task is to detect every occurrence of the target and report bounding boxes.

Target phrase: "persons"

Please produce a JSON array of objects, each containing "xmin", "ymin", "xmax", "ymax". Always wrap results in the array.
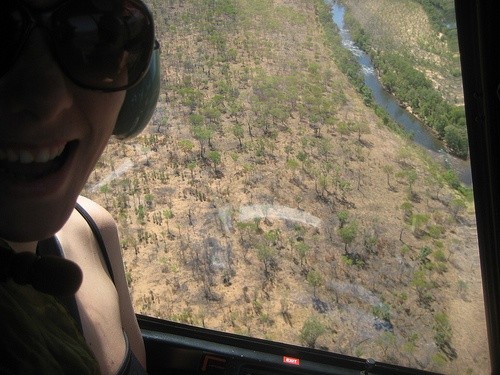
[{"xmin": 0, "ymin": 0, "xmax": 160, "ymax": 375}]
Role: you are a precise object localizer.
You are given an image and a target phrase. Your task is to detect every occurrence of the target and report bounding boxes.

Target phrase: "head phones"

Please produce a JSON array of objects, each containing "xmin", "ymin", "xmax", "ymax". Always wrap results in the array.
[{"xmin": 111, "ymin": 40, "xmax": 162, "ymax": 143}]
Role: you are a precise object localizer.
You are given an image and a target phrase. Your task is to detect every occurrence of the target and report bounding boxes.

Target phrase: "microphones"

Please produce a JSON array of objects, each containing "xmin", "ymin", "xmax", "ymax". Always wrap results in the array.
[{"xmin": 0, "ymin": 252, "xmax": 84, "ymax": 296}]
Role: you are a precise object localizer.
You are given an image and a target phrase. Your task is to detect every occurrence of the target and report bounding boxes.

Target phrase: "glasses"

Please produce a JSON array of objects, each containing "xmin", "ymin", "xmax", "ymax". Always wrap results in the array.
[{"xmin": 0, "ymin": 0, "xmax": 160, "ymax": 94}]
